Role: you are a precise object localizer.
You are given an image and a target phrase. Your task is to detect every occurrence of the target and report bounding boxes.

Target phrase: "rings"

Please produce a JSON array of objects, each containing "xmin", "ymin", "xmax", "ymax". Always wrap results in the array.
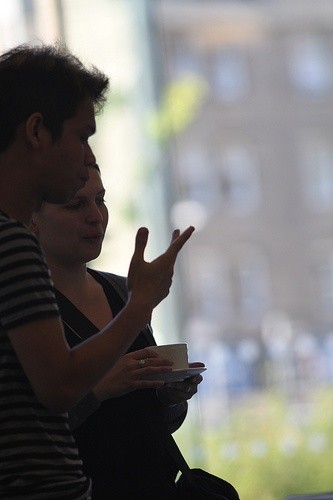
[{"xmin": 137, "ymin": 359, "xmax": 145, "ymax": 368}]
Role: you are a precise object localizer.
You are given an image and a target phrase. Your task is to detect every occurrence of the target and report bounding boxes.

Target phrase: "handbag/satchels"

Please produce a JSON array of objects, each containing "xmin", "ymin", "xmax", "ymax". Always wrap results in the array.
[{"xmin": 166, "ymin": 469, "xmax": 239, "ymax": 500}]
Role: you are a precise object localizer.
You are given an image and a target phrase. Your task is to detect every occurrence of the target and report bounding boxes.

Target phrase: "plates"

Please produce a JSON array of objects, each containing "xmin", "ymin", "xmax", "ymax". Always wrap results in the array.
[{"xmin": 135, "ymin": 367, "xmax": 207, "ymax": 385}]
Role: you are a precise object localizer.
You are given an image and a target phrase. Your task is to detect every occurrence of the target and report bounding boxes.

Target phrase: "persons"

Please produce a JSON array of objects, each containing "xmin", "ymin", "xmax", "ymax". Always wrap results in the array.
[
  {"xmin": 0, "ymin": 44, "xmax": 197, "ymax": 500},
  {"xmin": 24, "ymin": 163, "xmax": 206, "ymax": 500}
]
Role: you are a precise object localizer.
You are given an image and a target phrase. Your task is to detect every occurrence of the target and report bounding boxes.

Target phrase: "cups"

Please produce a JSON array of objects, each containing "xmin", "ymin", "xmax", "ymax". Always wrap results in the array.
[{"xmin": 145, "ymin": 344, "xmax": 189, "ymax": 369}]
